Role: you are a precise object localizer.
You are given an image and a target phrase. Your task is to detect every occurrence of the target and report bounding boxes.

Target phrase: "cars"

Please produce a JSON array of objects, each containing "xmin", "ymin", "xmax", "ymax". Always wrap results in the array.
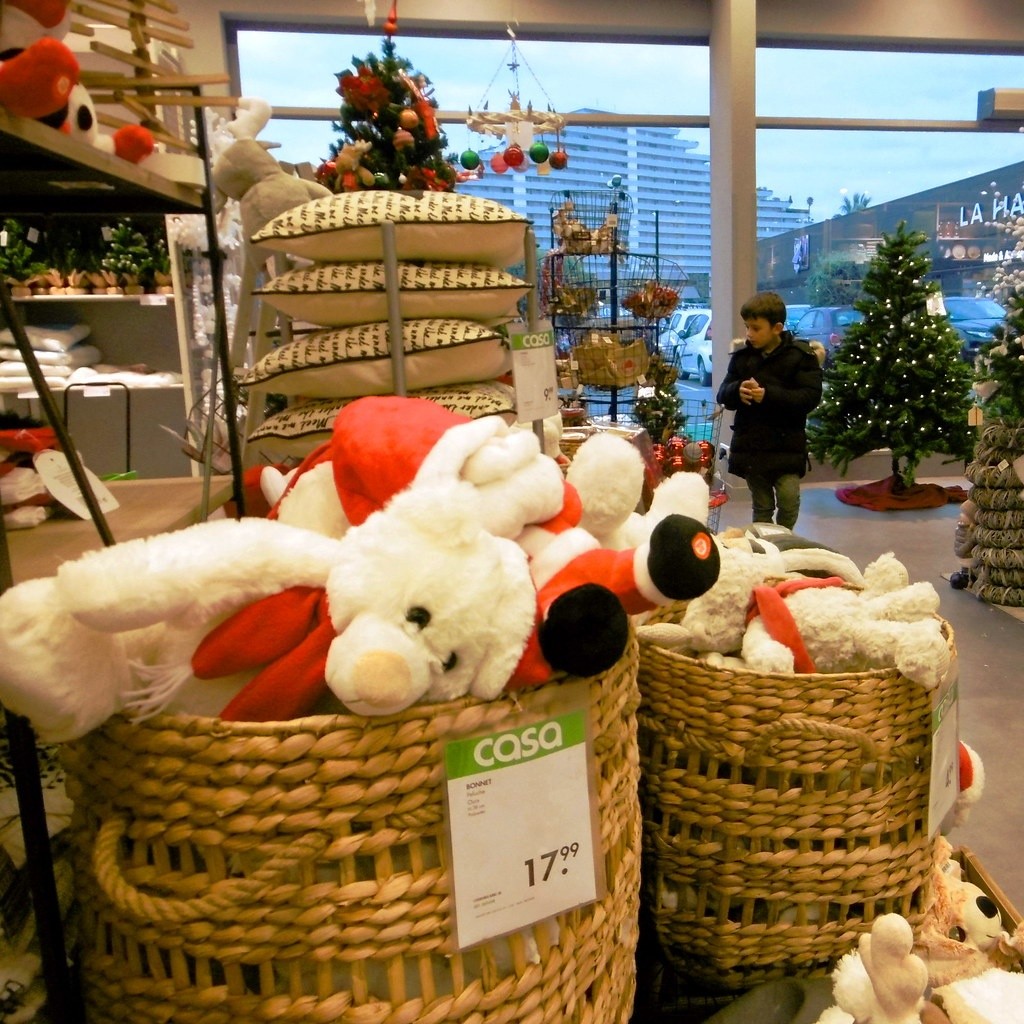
[
  {"xmin": 785, "ymin": 303, "xmax": 864, "ymax": 369},
  {"xmin": 944, "ymin": 297, "xmax": 1006, "ymax": 363},
  {"xmin": 656, "ymin": 309, "xmax": 712, "ymax": 387}
]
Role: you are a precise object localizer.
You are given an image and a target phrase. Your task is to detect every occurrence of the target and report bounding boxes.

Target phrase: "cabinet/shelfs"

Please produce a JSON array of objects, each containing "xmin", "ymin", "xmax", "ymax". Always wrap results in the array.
[{"xmin": 0, "ymin": 103, "xmax": 232, "ymax": 594}]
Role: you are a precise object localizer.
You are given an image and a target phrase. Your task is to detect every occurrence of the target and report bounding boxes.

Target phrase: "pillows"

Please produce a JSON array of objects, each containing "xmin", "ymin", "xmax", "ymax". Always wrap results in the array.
[
  {"xmin": 249, "ymin": 188, "xmax": 535, "ymax": 269},
  {"xmin": 237, "ymin": 320, "xmax": 514, "ymax": 401},
  {"xmin": 251, "ymin": 260, "xmax": 535, "ymax": 329},
  {"xmin": 245, "ymin": 380, "xmax": 516, "ymax": 461}
]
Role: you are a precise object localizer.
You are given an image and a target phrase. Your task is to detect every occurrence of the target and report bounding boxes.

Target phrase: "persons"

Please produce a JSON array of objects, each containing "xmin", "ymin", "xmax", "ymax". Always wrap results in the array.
[{"xmin": 716, "ymin": 291, "xmax": 823, "ymax": 531}]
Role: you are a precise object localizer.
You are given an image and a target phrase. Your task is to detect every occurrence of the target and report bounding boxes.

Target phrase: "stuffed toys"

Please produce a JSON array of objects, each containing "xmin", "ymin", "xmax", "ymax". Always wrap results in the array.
[
  {"xmin": 209, "ymin": 96, "xmax": 334, "ymax": 267},
  {"xmin": 813, "ymin": 837, "xmax": 1024, "ymax": 1024},
  {"xmin": 0, "ymin": 396, "xmax": 986, "ymax": 829},
  {"xmin": 333, "ymin": 139, "xmax": 375, "ymax": 192},
  {"xmin": 0, "ymin": 0, "xmax": 155, "ymax": 165}
]
